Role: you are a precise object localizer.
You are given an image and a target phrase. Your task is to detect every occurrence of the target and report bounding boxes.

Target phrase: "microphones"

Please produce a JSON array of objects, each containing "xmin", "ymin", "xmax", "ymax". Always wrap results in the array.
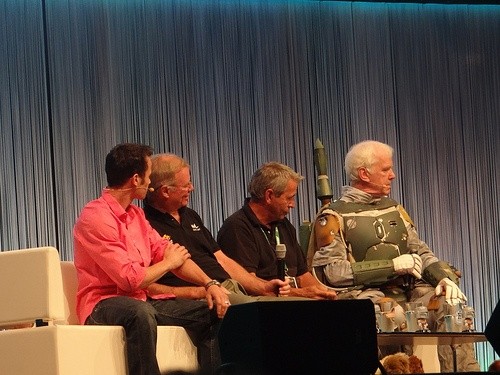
[{"xmin": 275, "ymin": 244, "xmax": 287, "ymax": 293}]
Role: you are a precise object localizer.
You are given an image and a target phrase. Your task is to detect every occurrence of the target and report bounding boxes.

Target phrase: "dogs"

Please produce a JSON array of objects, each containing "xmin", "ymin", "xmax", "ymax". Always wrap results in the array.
[{"xmin": 375, "ymin": 351, "xmax": 425, "ymax": 375}]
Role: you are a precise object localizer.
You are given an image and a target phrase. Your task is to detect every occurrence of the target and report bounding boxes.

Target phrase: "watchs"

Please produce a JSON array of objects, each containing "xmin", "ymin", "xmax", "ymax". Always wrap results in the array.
[{"xmin": 205, "ymin": 280, "xmax": 221, "ymax": 291}]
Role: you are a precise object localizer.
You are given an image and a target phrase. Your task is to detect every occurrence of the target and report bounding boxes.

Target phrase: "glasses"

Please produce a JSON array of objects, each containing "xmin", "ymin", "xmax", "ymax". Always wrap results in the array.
[{"xmin": 170, "ymin": 181, "xmax": 191, "ymax": 190}]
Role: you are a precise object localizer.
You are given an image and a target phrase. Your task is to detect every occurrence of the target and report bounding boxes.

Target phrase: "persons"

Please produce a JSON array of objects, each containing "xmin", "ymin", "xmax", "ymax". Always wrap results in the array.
[
  {"xmin": 73, "ymin": 143, "xmax": 232, "ymax": 375},
  {"xmin": 314, "ymin": 140, "xmax": 480, "ymax": 372},
  {"xmin": 217, "ymin": 161, "xmax": 336, "ymax": 299},
  {"xmin": 144, "ymin": 154, "xmax": 317, "ymax": 304}
]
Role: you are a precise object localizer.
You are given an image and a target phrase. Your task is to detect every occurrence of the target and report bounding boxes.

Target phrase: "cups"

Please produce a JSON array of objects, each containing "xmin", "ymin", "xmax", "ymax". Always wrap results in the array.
[
  {"xmin": 374, "ymin": 300, "xmax": 429, "ymax": 333},
  {"xmin": 444, "ymin": 303, "xmax": 474, "ymax": 333}
]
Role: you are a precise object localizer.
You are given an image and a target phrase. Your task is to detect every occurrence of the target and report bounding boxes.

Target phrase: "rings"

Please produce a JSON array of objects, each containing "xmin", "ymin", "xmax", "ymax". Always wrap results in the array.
[{"xmin": 225, "ymin": 301, "xmax": 229, "ymax": 304}]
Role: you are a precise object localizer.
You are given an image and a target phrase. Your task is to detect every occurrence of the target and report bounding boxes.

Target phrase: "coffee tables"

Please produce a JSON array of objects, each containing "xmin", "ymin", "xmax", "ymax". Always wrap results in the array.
[{"xmin": 377, "ymin": 332, "xmax": 488, "ymax": 373}]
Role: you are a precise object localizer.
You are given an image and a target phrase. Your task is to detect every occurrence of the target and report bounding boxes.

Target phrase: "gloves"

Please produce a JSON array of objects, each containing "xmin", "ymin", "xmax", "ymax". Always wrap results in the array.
[
  {"xmin": 392, "ymin": 253, "xmax": 422, "ymax": 279},
  {"xmin": 435, "ymin": 278, "xmax": 467, "ymax": 303}
]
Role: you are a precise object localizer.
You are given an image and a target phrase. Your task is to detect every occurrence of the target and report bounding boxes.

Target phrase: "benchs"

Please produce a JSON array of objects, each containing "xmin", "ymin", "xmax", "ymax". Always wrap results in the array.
[{"xmin": 0, "ymin": 247, "xmax": 442, "ymax": 375}]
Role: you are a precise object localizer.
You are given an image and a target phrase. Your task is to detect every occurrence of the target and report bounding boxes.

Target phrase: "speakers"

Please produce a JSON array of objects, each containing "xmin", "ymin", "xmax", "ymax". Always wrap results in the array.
[
  {"xmin": 484, "ymin": 301, "xmax": 500, "ymax": 357},
  {"xmin": 218, "ymin": 298, "xmax": 379, "ymax": 375}
]
